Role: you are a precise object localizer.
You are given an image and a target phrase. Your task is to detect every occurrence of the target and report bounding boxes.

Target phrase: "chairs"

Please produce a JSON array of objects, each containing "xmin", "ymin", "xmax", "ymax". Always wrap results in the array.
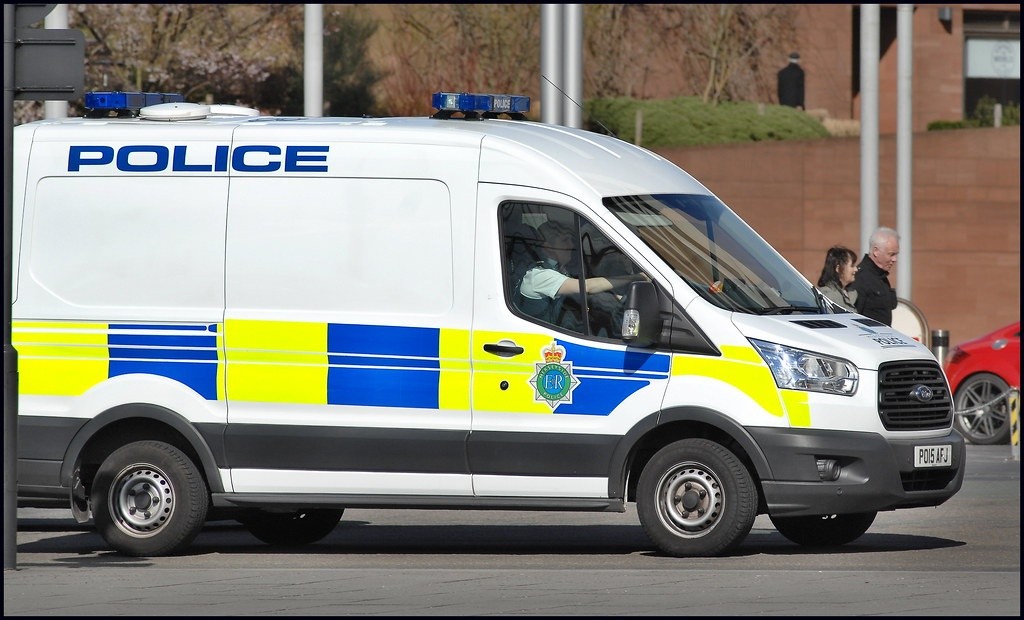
[{"xmin": 511, "ymin": 225, "xmax": 535, "ymax": 293}]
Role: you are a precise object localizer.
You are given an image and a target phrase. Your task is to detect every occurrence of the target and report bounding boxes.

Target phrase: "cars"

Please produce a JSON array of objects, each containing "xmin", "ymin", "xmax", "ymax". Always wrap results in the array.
[{"xmin": 940, "ymin": 320, "xmax": 1020, "ymax": 446}]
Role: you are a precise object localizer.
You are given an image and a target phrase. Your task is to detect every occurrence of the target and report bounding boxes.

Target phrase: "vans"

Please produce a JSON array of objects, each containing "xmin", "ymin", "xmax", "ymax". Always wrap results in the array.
[{"xmin": 11, "ymin": 91, "xmax": 968, "ymax": 559}]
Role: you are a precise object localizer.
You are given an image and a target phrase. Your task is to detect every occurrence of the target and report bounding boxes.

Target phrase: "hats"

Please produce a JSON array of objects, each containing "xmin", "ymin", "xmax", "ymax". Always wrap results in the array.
[{"xmin": 789, "ymin": 52, "xmax": 799, "ymax": 58}]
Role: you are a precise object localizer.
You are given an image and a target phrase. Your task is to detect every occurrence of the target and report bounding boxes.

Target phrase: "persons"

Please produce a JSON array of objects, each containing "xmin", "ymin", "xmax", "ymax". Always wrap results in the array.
[
  {"xmin": 844, "ymin": 227, "xmax": 900, "ymax": 328},
  {"xmin": 513, "ymin": 221, "xmax": 654, "ymax": 339},
  {"xmin": 777, "ymin": 52, "xmax": 806, "ymax": 112},
  {"xmin": 817, "ymin": 247, "xmax": 859, "ymax": 311}
]
